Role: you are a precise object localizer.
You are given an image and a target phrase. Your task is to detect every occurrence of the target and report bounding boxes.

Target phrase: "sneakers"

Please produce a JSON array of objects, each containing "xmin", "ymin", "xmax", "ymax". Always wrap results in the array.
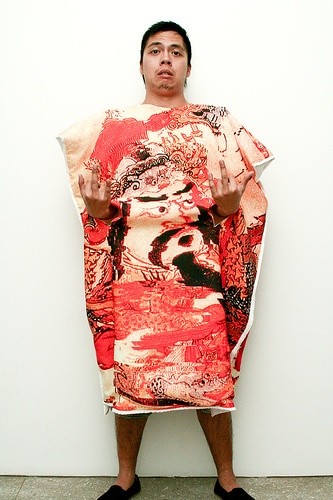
[
  {"xmin": 95, "ymin": 473, "xmax": 141, "ymax": 499},
  {"xmin": 214, "ymin": 479, "xmax": 255, "ymax": 500}
]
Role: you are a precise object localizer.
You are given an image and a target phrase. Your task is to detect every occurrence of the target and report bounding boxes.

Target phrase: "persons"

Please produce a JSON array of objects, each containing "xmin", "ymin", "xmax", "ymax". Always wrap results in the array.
[{"xmin": 55, "ymin": 21, "xmax": 273, "ymax": 500}]
